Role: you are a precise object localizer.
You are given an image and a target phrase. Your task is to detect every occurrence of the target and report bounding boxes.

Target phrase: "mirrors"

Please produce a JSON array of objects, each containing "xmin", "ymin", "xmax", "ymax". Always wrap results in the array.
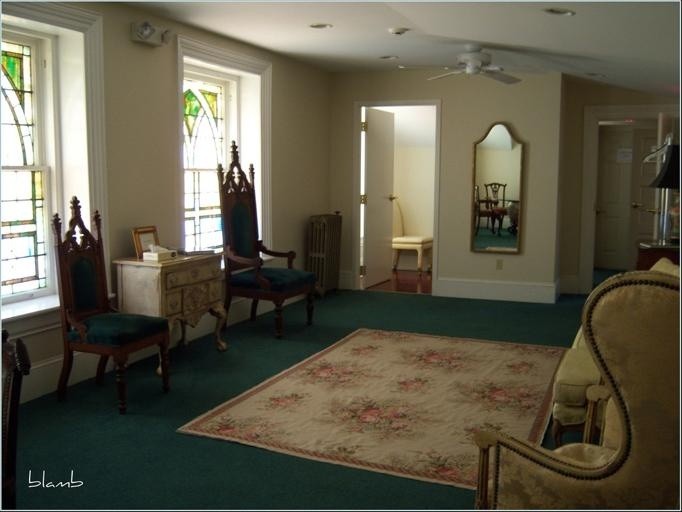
[{"xmin": 472, "ymin": 122, "xmax": 524, "ymax": 253}]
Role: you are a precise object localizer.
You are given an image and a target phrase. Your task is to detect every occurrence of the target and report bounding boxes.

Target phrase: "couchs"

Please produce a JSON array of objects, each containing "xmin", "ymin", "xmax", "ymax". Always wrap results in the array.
[{"xmin": 471, "ymin": 258, "xmax": 679, "ymax": 510}]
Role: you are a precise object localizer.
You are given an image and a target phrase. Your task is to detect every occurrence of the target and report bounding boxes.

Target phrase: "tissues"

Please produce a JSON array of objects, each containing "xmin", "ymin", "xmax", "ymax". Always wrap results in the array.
[{"xmin": 143, "ymin": 244, "xmax": 178, "ymax": 262}]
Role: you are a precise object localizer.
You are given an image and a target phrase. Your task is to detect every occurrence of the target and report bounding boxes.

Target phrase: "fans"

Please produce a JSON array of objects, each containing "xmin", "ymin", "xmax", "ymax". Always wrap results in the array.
[{"xmin": 424, "ymin": 54, "xmax": 522, "ymax": 85}]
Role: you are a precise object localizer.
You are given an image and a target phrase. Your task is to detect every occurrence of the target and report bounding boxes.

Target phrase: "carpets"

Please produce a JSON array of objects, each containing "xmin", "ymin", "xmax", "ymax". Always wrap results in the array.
[{"xmin": 176, "ymin": 327, "xmax": 571, "ymax": 491}]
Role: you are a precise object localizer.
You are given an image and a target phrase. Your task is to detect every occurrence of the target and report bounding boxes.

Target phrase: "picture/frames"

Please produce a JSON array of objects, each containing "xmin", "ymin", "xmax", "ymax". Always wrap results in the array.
[{"xmin": 130, "ymin": 225, "xmax": 160, "ymax": 260}]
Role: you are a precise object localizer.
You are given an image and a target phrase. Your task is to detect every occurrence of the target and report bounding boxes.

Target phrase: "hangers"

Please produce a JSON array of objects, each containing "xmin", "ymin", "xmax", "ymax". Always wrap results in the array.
[{"xmin": 641, "ymin": 130, "xmax": 674, "ymax": 164}]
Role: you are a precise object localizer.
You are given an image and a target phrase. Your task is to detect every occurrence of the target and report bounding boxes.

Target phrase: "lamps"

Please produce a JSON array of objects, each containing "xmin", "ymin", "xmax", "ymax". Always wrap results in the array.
[
  {"xmin": 640, "ymin": 143, "xmax": 679, "ymax": 247},
  {"xmin": 136, "ymin": 21, "xmax": 154, "ymax": 39},
  {"xmin": 456, "ymin": 40, "xmax": 491, "ymax": 78},
  {"xmin": 161, "ymin": 29, "xmax": 171, "ymax": 45}
]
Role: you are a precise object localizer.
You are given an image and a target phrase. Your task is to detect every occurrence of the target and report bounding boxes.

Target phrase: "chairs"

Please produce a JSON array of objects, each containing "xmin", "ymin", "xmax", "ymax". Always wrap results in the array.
[
  {"xmin": 392, "ymin": 196, "xmax": 432, "ymax": 274},
  {"xmin": 2, "ymin": 330, "xmax": 30, "ymax": 506},
  {"xmin": 216, "ymin": 140, "xmax": 320, "ymax": 340},
  {"xmin": 50, "ymin": 196, "xmax": 171, "ymax": 415},
  {"xmin": 473, "ymin": 183, "xmax": 520, "ymax": 237}
]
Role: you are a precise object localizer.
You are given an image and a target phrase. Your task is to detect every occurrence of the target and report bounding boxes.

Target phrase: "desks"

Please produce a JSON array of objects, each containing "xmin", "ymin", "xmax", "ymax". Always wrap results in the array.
[
  {"xmin": 637, "ymin": 238, "xmax": 679, "ymax": 271},
  {"xmin": 111, "ymin": 250, "xmax": 228, "ymax": 373}
]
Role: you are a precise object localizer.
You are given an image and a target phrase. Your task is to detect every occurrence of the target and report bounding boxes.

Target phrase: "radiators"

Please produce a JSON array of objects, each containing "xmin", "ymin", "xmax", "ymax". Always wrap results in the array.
[{"xmin": 306, "ymin": 210, "xmax": 342, "ymax": 296}]
